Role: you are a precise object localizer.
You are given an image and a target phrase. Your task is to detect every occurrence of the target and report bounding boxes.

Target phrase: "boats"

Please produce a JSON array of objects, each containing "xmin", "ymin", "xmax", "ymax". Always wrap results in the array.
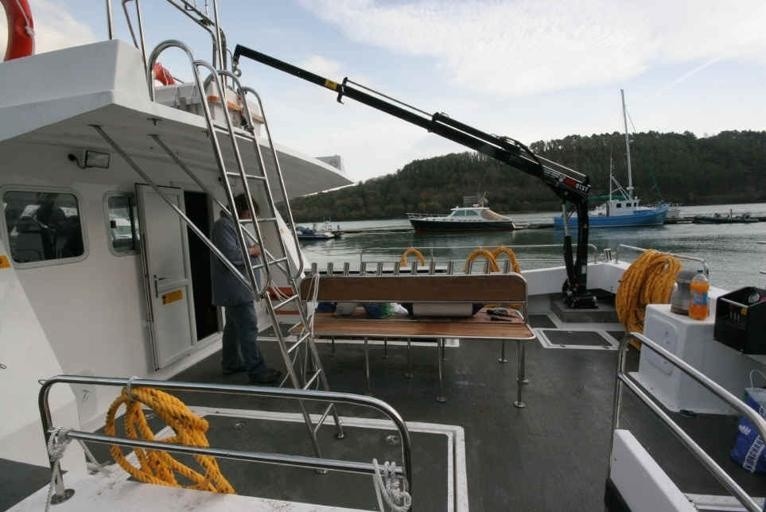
[
  {"xmin": 296, "ymin": 216, "xmax": 344, "ymax": 240},
  {"xmin": 692, "ymin": 215, "xmax": 766, "ymax": 224},
  {"xmin": 404, "ymin": 192, "xmax": 516, "ymax": 237},
  {"xmin": 641, "ymin": 200, "xmax": 680, "ymax": 224}
]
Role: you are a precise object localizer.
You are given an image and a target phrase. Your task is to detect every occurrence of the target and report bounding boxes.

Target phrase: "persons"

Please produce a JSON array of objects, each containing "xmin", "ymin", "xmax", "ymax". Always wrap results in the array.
[{"xmin": 210, "ymin": 193, "xmax": 283, "ymax": 382}]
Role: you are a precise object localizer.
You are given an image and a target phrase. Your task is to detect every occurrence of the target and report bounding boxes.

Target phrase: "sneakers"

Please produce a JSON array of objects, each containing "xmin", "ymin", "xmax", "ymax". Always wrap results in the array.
[{"xmin": 221, "ymin": 359, "xmax": 284, "ymax": 385}]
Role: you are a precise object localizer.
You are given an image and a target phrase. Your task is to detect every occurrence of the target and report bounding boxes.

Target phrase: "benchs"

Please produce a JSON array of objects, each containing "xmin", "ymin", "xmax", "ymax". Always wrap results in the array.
[{"xmin": 288, "ymin": 275, "xmax": 537, "ymax": 411}]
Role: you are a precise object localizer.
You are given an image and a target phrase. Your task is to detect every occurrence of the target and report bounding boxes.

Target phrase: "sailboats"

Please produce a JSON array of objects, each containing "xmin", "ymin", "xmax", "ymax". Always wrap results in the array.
[{"xmin": 554, "ymin": 89, "xmax": 668, "ymax": 230}]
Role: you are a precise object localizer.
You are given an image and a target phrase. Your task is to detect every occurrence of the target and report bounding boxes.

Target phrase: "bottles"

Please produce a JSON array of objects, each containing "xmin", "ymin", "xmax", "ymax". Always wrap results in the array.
[{"xmin": 689, "ymin": 270, "xmax": 710, "ymax": 320}]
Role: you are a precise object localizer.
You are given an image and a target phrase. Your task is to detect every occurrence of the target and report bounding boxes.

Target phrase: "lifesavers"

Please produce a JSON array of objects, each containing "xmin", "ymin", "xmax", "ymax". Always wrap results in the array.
[
  {"xmin": 0, "ymin": 0, "xmax": 34, "ymax": 62},
  {"xmin": 155, "ymin": 64, "xmax": 175, "ymax": 86}
]
